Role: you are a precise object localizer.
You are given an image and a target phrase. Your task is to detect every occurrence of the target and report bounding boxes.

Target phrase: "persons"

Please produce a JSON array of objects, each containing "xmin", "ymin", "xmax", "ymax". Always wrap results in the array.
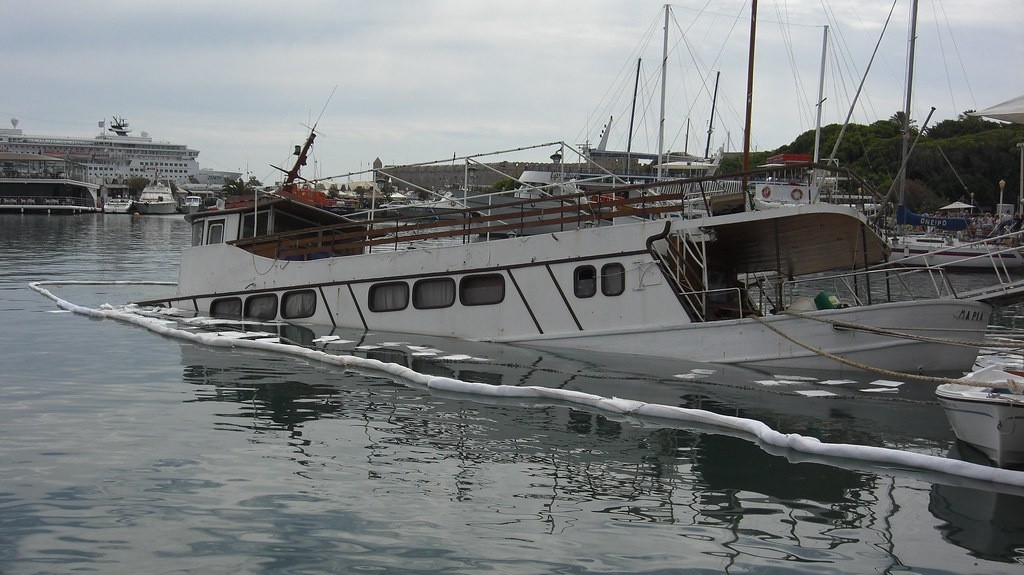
[{"xmin": 922, "ymin": 210, "xmax": 1024, "ymax": 229}]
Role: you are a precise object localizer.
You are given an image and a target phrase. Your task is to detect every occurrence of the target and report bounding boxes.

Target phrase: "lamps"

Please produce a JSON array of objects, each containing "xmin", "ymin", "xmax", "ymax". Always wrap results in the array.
[{"xmin": 550, "ymin": 147, "xmax": 562, "ymax": 165}]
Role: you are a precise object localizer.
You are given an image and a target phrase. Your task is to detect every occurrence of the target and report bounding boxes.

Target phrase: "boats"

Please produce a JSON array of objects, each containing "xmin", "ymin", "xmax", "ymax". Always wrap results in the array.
[{"xmin": 935, "ymin": 360, "xmax": 1023, "ymax": 470}]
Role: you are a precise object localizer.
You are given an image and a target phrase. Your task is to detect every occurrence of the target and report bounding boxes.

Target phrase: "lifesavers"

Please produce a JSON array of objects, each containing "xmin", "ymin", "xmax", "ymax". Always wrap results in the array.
[
  {"xmin": 791, "ymin": 188, "xmax": 803, "ymax": 201},
  {"xmin": 761, "ymin": 186, "xmax": 772, "ymax": 198}
]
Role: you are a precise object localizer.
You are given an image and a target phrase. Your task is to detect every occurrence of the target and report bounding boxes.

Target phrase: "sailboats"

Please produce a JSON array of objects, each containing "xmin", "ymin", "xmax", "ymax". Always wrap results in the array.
[{"xmin": 103, "ymin": 0, "xmax": 1024, "ymax": 376}]
